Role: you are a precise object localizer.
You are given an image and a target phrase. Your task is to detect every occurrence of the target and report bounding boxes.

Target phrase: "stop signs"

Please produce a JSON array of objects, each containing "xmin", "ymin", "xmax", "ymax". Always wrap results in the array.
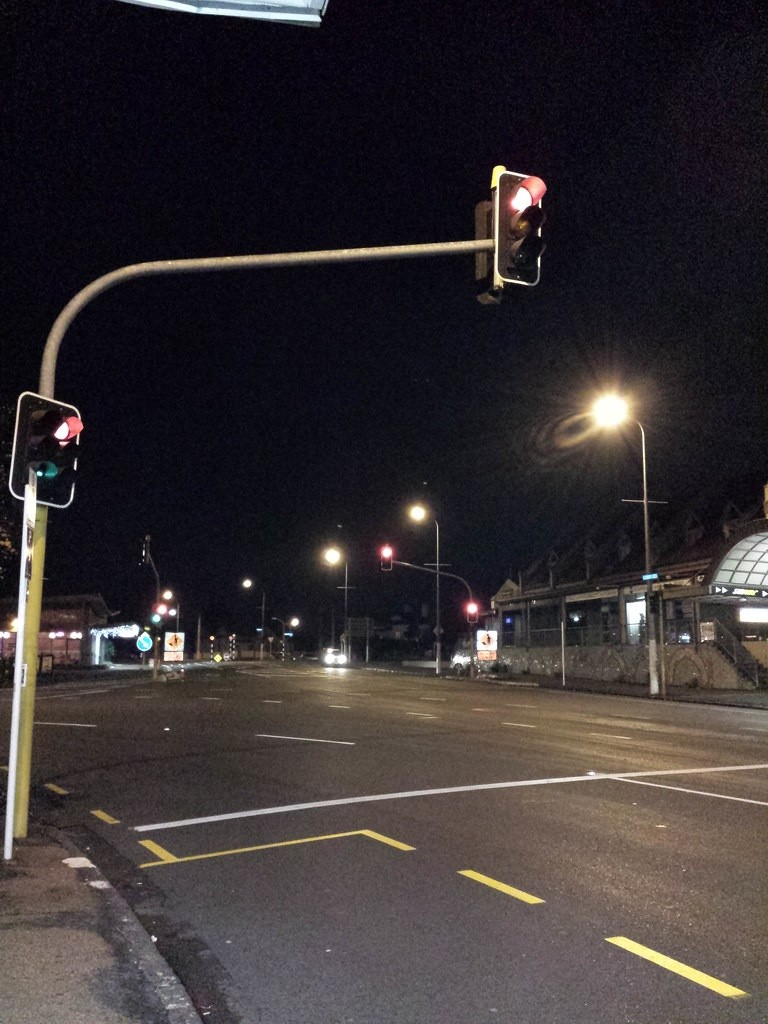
[{"xmin": 225, "ymin": 654, "xmax": 229, "ymax": 660}]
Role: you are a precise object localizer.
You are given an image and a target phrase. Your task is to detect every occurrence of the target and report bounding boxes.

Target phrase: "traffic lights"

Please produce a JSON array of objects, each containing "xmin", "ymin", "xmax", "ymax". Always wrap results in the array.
[
  {"xmin": 140, "ymin": 536, "xmax": 149, "ymax": 562},
  {"xmin": 6, "ymin": 390, "xmax": 83, "ymax": 509},
  {"xmin": 467, "ymin": 603, "xmax": 478, "ymax": 624},
  {"xmin": 154, "ymin": 605, "xmax": 166, "ymax": 625},
  {"xmin": 380, "ymin": 546, "xmax": 392, "ymax": 571},
  {"xmin": 494, "ymin": 169, "xmax": 550, "ymax": 286}
]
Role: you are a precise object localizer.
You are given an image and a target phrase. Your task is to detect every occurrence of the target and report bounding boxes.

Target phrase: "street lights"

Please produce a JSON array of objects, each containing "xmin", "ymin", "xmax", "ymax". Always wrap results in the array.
[
  {"xmin": 0, "ymin": 632, "xmax": 11, "ymax": 655},
  {"xmin": 289, "ymin": 618, "xmax": 300, "ymax": 659},
  {"xmin": 272, "ymin": 618, "xmax": 286, "ymax": 660},
  {"xmin": 164, "ymin": 591, "xmax": 180, "ymax": 632},
  {"xmin": 324, "ymin": 546, "xmax": 348, "ymax": 664},
  {"xmin": 244, "ymin": 580, "xmax": 265, "ymax": 660},
  {"xmin": 593, "ymin": 395, "xmax": 659, "ymax": 696},
  {"xmin": 411, "ymin": 506, "xmax": 441, "ymax": 677}
]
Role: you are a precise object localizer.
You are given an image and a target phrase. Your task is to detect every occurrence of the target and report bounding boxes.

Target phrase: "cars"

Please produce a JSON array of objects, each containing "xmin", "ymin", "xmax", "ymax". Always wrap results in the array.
[
  {"xmin": 451, "ymin": 650, "xmax": 495, "ymax": 676},
  {"xmin": 324, "ymin": 649, "xmax": 347, "ymax": 667}
]
